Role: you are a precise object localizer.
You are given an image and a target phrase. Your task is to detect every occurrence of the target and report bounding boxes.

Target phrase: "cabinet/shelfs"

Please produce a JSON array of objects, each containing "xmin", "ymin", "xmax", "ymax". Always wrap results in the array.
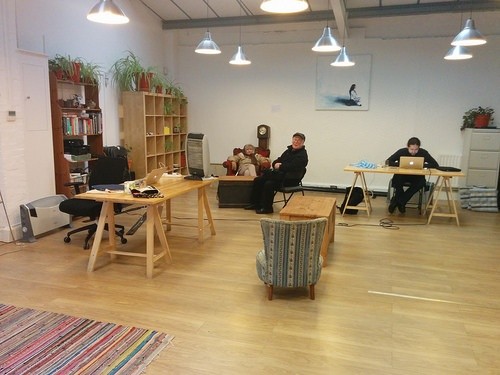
[
  {"xmin": 49, "ymin": 72, "xmax": 105, "ymax": 198},
  {"xmin": 121, "ymin": 91, "xmax": 189, "ymax": 179},
  {"xmin": 461, "ymin": 126, "xmax": 500, "ymax": 210}
]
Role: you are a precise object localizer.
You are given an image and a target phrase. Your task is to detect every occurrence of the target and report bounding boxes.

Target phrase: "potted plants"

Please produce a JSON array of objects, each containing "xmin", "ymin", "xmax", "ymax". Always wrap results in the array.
[
  {"xmin": 49, "ymin": 54, "xmax": 84, "ymax": 82},
  {"xmin": 461, "ymin": 107, "xmax": 492, "ymax": 129},
  {"xmin": 115, "ymin": 51, "xmax": 173, "ymax": 94}
]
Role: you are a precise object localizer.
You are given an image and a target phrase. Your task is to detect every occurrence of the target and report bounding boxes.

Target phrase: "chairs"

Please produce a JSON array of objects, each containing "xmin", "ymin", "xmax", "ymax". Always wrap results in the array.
[
  {"xmin": 274, "ymin": 168, "xmax": 307, "ymax": 209},
  {"xmin": 256, "ymin": 216, "xmax": 330, "ymax": 300},
  {"xmin": 388, "ymin": 181, "xmax": 425, "ymax": 216},
  {"xmin": 56, "ymin": 158, "xmax": 128, "ymax": 249}
]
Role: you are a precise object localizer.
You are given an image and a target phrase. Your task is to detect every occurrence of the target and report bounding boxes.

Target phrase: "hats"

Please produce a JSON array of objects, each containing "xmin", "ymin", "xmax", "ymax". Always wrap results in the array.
[{"xmin": 293, "ymin": 132, "xmax": 305, "ymax": 142}]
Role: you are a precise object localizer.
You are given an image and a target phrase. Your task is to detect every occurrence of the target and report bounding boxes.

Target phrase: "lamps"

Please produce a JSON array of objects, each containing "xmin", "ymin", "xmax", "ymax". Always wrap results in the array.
[
  {"xmin": 87, "ymin": 0, "xmax": 129, "ymax": 25},
  {"xmin": 331, "ymin": 10, "xmax": 355, "ymax": 67},
  {"xmin": 228, "ymin": 3, "xmax": 251, "ymax": 66},
  {"xmin": 311, "ymin": 0, "xmax": 341, "ymax": 52},
  {"xmin": 450, "ymin": 8, "xmax": 486, "ymax": 47},
  {"xmin": 194, "ymin": 0, "xmax": 222, "ymax": 54},
  {"xmin": 444, "ymin": 12, "xmax": 472, "ymax": 60}
]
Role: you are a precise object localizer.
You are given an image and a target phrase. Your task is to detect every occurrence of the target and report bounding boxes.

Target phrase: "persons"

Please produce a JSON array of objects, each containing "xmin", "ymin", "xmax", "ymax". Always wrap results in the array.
[
  {"xmin": 245, "ymin": 133, "xmax": 308, "ymax": 214},
  {"xmin": 385, "ymin": 137, "xmax": 439, "ymax": 214},
  {"xmin": 227, "ymin": 144, "xmax": 271, "ymax": 177}
]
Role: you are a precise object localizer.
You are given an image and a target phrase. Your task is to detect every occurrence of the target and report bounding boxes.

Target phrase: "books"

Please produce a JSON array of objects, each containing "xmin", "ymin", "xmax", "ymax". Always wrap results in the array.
[{"xmin": 62, "ymin": 111, "xmax": 102, "ymax": 136}]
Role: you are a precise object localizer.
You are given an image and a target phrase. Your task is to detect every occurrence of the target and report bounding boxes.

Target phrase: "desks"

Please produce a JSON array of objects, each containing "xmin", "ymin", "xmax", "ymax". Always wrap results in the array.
[
  {"xmin": 218, "ymin": 176, "xmax": 256, "ymax": 208},
  {"xmin": 279, "ymin": 196, "xmax": 337, "ymax": 266},
  {"xmin": 342, "ymin": 166, "xmax": 464, "ymax": 225},
  {"xmin": 73, "ymin": 175, "xmax": 217, "ymax": 279}
]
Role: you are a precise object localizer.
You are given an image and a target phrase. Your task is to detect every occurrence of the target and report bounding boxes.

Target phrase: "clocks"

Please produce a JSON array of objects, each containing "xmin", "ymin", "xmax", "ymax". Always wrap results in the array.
[{"xmin": 257, "ymin": 124, "xmax": 271, "ymax": 147}]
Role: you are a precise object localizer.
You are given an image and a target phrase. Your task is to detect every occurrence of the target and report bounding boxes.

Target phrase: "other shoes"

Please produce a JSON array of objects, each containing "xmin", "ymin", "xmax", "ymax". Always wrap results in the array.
[
  {"xmin": 244, "ymin": 204, "xmax": 257, "ymax": 210},
  {"xmin": 256, "ymin": 208, "xmax": 273, "ymax": 214},
  {"xmin": 397, "ymin": 203, "xmax": 405, "ymax": 214},
  {"xmin": 388, "ymin": 199, "xmax": 398, "ymax": 214}
]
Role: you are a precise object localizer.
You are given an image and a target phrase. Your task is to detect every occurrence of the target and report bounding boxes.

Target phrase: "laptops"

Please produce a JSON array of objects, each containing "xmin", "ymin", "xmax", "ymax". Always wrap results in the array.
[{"xmin": 399, "ymin": 156, "xmax": 424, "ymax": 170}]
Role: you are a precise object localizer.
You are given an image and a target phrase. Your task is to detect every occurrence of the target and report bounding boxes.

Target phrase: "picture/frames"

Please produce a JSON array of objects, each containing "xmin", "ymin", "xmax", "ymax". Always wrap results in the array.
[{"xmin": 315, "ymin": 53, "xmax": 372, "ymax": 112}]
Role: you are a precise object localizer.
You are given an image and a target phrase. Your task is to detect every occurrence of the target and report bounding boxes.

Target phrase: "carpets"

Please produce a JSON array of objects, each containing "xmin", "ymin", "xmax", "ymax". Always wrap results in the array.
[{"xmin": 0, "ymin": 303, "xmax": 176, "ymax": 375}]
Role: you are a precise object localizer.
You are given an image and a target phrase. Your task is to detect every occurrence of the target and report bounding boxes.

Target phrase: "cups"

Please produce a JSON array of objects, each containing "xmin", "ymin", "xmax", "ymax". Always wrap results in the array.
[{"xmin": 124, "ymin": 181, "xmax": 134, "ymax": 194}]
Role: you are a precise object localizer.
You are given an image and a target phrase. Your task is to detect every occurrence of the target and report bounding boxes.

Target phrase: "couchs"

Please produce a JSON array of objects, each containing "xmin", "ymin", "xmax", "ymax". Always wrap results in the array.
[{"xmin": 222, "ymin": 148, "xmax": 270, "ymax": 176}]
